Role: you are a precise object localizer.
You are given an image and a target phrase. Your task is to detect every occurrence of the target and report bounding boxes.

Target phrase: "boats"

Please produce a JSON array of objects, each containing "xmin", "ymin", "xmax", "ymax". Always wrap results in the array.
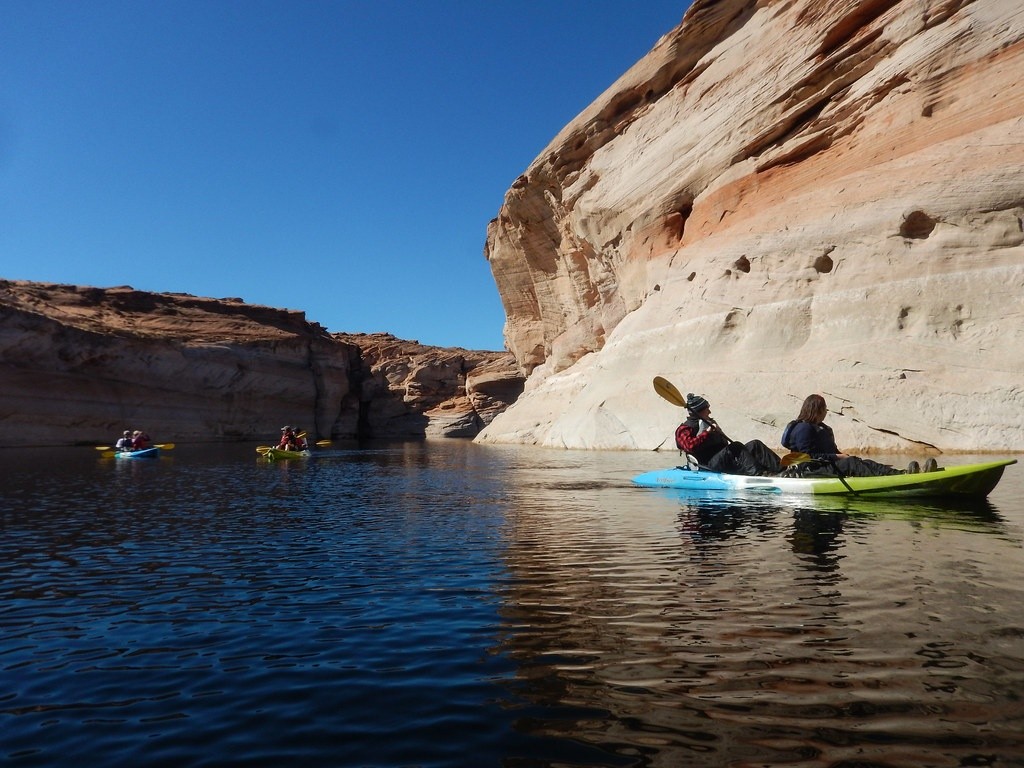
[
  {"xmin": 632, "ymin": 457, "xmax": 1019, "ymax": 500},
  {"xmin": 256, "ymin": 443, "xmax": 312, "ymax": 459},
  {"xmin": 113, "ymin": 446, "xmax": 161, "ymax": 459}
]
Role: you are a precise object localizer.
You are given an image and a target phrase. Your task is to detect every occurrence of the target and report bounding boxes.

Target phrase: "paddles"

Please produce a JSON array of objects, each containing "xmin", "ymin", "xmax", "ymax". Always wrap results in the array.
[
  {"xmin": 780, "ymin": 453, "xmax": 894, "ymax": 470},
  {"xmin": 263, "ymin": 431, "xmax": 309, "ymax": 456},
  {"xmin": 256, "ymin": 440, "xmax": 332, "ymax": 454},
  {"xmin": 652, "ymin": 375, "xmax": 736, "ymax": 443},
  {"xmin": 95, "ymin": 443, "xmax": 175, "ymax": 451}
]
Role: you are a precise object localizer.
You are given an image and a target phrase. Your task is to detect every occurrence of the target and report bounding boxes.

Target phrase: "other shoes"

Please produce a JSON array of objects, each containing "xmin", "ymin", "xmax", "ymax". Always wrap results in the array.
[
  {"xmin": 907, "ymin": 461, "xmax": 919, "ymax": 474},
  {"xmin": 921, "ymin": 459, "xmax": 937, "ymax": 472}
]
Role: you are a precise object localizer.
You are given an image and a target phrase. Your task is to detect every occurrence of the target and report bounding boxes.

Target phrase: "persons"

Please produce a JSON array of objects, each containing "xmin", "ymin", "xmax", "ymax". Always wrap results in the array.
[
  {"xmin": 791, "ymin": 394, "xmax": 937, "ymax": 475},
  {"xmin": 116, "ymin": 430, "xmax": 151, "ymax": 451},
  {"xmin": 276, "ymin": 426, "xmax": 308, "ymax": 451},
  {"xmin": 675, "ymin": 392, "xmax": 785, "ymax": 475}
]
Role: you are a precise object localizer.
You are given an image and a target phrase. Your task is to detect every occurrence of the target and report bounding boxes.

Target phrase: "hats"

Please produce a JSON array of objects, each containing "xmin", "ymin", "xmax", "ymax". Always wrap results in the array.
[
  {"xmin": 133, "ymin": 431, "xmax": 142, "ymax": 438},
  {"xmin": 291, "ymin": 427, "xmax": 300, "ymax": 433},
  {"xmin": 281, "ymin": 426, "xmax": 291, "ymax": 431},
  {"xmin": 687, "ymin": 393, "xmax": 709, "ymax": 415},
  {"xmin": 124, "ymin": 430, "xmax": 130, "ymax": 435}
]
